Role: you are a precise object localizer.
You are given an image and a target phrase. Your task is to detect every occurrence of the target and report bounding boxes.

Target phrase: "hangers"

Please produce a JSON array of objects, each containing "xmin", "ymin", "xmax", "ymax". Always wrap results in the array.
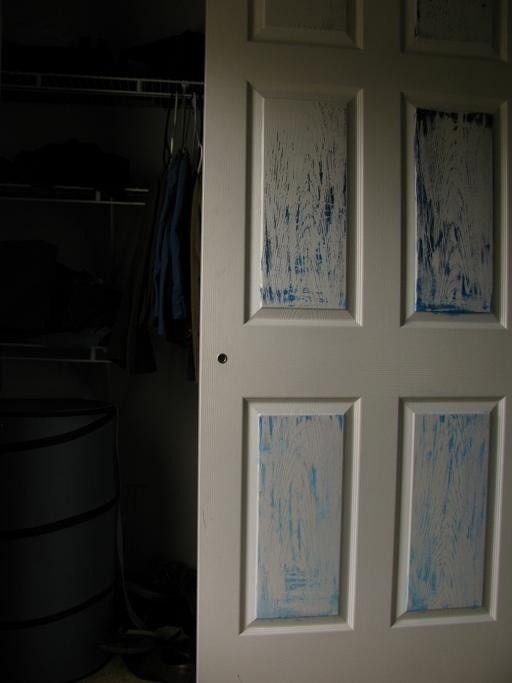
[{"xmin": 161, "ymin": 90, "xmax": 202, "ymax": 177}]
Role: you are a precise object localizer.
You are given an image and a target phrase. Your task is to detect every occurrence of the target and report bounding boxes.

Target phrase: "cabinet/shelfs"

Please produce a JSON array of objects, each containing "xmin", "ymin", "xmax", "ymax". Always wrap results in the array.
[{"xmin": 2, "ymin": 70, "xmax": 202, "ymax": 367}]
